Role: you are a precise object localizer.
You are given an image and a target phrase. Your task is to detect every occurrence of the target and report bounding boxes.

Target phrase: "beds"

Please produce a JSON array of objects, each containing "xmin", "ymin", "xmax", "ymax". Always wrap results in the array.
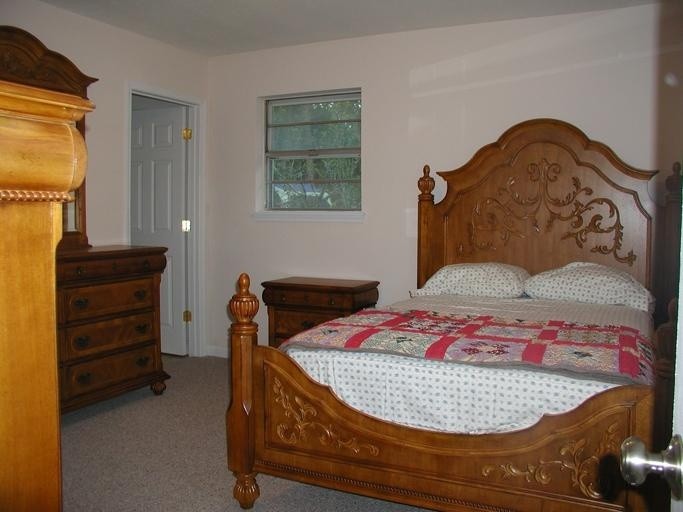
[{"xmin": 225, "ymin": 117, "xmax": 682, "ymax": 511}]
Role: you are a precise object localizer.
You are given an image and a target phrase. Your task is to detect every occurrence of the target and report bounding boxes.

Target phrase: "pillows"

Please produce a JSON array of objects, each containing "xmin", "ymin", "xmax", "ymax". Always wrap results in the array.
[{"xmin": 406, "ymin": 260, "xmax": 660, "ymax": 316}]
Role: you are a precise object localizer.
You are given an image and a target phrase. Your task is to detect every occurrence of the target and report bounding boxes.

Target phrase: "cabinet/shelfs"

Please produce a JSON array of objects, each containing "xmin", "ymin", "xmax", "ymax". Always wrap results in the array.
[
  {"xmin": 260, "ymin": 275, "xmax": 380, "ymax": 348},
  {"xmin": 54, "ymin": 230, "xmax": 171, "ymax": 415}
]
